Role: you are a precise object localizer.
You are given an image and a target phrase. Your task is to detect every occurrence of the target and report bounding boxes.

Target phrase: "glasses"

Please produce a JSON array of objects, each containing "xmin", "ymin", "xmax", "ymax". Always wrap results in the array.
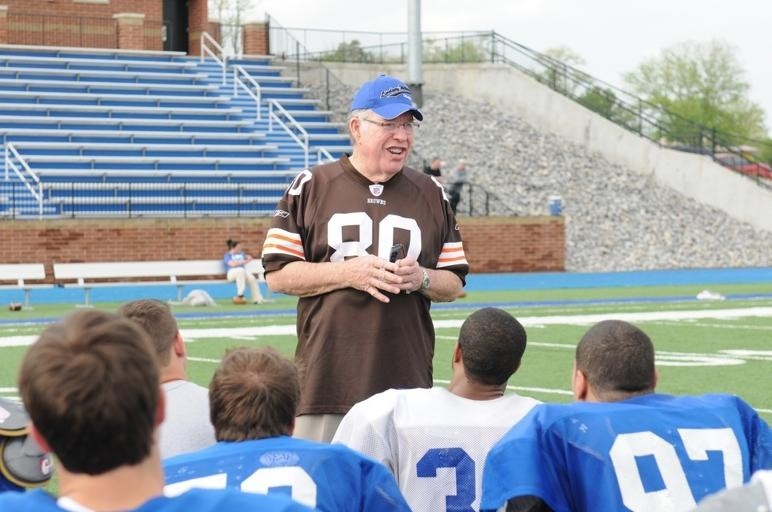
[{"xmin": 356, "ymin": 117, "xmax": 419, "ymax": 134}]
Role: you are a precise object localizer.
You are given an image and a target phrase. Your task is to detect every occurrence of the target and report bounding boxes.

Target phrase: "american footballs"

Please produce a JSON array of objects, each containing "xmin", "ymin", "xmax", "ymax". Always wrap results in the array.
[{"xmin": 233, "ymin": 296, "xmax": 246, "ymax": 304}]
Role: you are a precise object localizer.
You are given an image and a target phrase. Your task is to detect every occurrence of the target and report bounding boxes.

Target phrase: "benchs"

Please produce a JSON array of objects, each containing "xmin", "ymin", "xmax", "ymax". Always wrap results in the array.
[
  {"xmin": 0, "ymin": 261, "xmax": 46, "ymax": 309},
  {"xmin": 53, "ymin": 258, "xmax": 264, "ymax": 306}
]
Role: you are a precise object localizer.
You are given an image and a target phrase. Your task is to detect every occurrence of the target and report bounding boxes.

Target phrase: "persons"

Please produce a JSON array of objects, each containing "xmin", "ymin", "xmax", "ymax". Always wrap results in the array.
[
  {"xmin": 262, "ymin": 73, "xmax": 470, "ymax": 444},
  {"xmin": 444, "ymin": 159, "xmax": 469, "ymax": 216},
  {"xmin": 423, "ymin": 157, "xmax": 449, "ymax": 183},
  {"xmin": 221, "ymin": 239, "xmax": 262, "ymax": 304},
  {"xmin": 159, "ymin": 342, "xmax": 413, "ymax": 511},
  {"xmin": 1, "ymin": 306, "xmax": 321, "ymax": 511},
  {"xmin": 330, "ymin": 304, "xmax": 545, "ymax": 511},
  {"xmin": 479, "ymin": 319, "xmax": 772, "ymax": 512},
  {"xmin": 117, "ymin": 295, "xmax": 217, "ymax": 461}
]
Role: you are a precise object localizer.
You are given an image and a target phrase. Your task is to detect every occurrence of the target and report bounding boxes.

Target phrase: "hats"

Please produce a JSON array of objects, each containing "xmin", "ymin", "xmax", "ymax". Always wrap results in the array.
[{"xmin": 351, "ymin": 74, "xmax": 423, "ymax": 121}]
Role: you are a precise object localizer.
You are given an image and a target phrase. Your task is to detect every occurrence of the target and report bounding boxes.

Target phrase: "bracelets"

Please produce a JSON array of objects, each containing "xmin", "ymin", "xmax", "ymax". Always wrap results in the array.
[{"xmin": 418, "ymin": 267, "xmax": 431, "ymax": 295}]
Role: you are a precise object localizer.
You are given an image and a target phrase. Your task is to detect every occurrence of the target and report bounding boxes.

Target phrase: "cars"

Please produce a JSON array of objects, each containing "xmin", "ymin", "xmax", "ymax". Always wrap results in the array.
[{"xmin": 718, "ymin": 153, "xmax": 772, "ymax": 180}]
